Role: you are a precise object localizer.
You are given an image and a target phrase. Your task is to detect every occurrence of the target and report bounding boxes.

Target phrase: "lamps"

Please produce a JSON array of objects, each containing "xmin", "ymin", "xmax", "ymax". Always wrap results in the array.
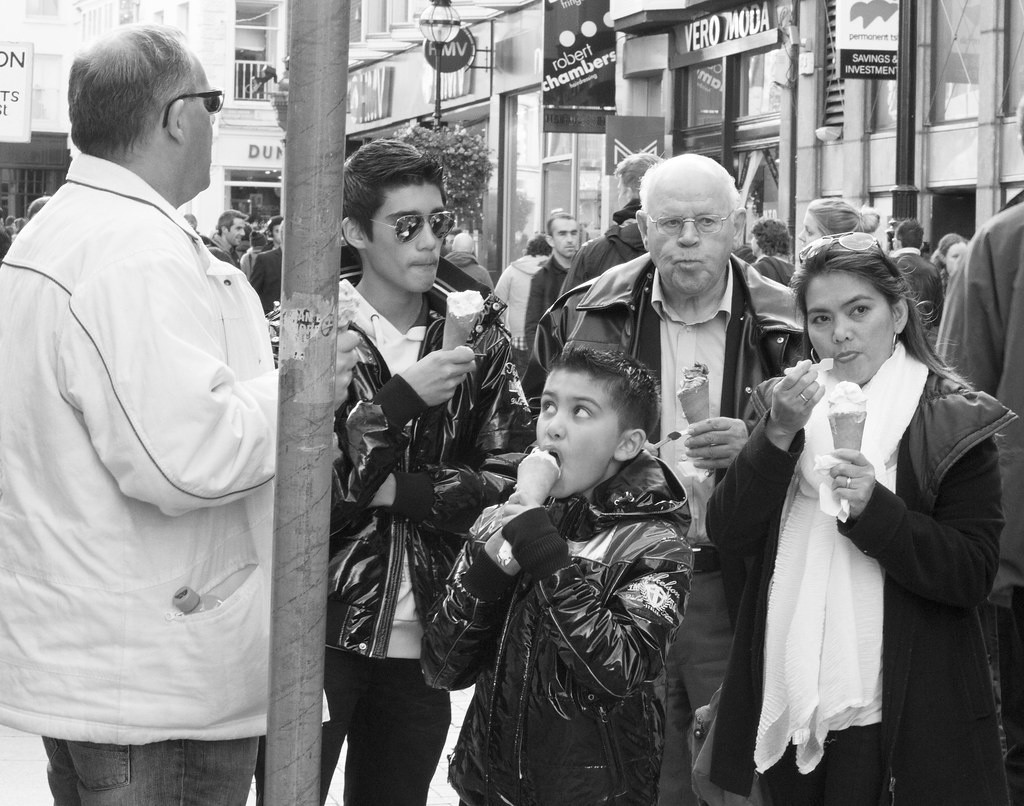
[
  {"xmin": 815, "ymin": 126, "xmax": 843, "ymax": 142},
  {"xmin": 252, "ymin": 66, "xmax": 278, "ymax": 92}
]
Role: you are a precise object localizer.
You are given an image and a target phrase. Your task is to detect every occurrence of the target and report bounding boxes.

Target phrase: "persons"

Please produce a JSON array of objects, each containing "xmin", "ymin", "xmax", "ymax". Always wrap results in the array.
[
  {"xmin": 495, "ymin": 234, "xmax": 553, "ymax": 378},
  {"xmin": 559, "ymin": 153, "xmax": 665, "ymax": 296},
  {"xmin": 750, "ymin": 218, "xmax": 796, "ymax": 287},
  {"xmin": 420, "ymin": 345, "xmax": 696, "ymax": 806},
  {"xmin": 521, "ymin": 153, "xmax": 805, "ymax": 806},
  {"xmin": 253, "ymin": 139, "xmax": 532, "ymax": 806},
  {"xmin": 443, "ymin": 233, "xmax": 494, "ymax": 293},
  {"xmin": 798, "ymin": 199, "xmax": 881, "ymax": 247},
  {"xmin": 184, "ymin": 209, "xmax": 284, "ymax": 314},
  {"xmin": 888, "ymin": 218, "xmax": 969, "ymax": 330},
  {"xmin": 525, "ymin": 213, "xmax": 583, "ymax": 347},
  {"xmin": 936, "ymin": 198, "xmax": 1024, "ymax": 806},
  {"xmin": 0, "ymin": 196, "xmax": 52, "ymax": 266},
  {"xmin": 0, "ymin": 23, "xmax": 360, "ymax": 806},
  {"xmin": 707, "ymin": 232, "xmax": 1020, "ymax": 806}
]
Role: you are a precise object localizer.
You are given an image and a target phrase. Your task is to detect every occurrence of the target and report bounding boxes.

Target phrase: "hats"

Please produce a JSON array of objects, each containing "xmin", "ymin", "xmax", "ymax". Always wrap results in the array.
[{"xmin": 249, "ymin": 231, "xmax": 266, "ymax": 246}]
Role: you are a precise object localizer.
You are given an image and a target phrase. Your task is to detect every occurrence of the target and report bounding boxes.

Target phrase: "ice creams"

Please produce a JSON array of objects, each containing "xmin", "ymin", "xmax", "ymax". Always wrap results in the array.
[
  {"xmin": 442, "ymin": 289, "xmax": 484, "ymax": 351},
  {"xmin": 337, "ymin": 283, "xmax": 360, "ymax": 334},
  {"xmin": 676, "ymin": 361, "xmax": 714, "ymax": 474},
  {"xmin": 517, "ymin": 449, "xmax": 561, "ymax": 508},
  {"xmin": 827, "ymin": 380, "xmax": 867, "ymax": 455}
]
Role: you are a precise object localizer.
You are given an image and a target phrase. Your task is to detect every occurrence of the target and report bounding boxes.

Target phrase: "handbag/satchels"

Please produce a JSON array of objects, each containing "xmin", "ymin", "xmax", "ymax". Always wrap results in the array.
[{"xmin": 690, "ymin": 683, "xmax": 775, "ymax": 806}]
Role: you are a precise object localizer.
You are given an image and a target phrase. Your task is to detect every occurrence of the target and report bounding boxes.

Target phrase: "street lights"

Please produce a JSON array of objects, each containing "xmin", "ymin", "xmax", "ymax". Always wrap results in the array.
[{"xmin": 420, "ymin": 0, "xmax": 460, "ymax": 132}]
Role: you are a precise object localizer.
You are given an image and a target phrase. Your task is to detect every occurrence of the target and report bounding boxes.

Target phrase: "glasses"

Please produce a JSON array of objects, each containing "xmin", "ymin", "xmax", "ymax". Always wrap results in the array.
[
  {"xmin": 369, "ymin": 206, "xmax": 455, "ymax": 243},
  {"xmin": 798, "ymin": 231, "xmax": 900, "ymax": 267},
  {"xmin": 162, "ymin": 88, "xmax": 226, "ymax": 129},
  {"xmin": 646, "ymin": 208, "xmax": 736, "ymax": 238}
]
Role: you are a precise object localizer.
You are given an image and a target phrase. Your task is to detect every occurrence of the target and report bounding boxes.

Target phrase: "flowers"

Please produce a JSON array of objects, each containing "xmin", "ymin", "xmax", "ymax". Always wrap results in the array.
[{"xmin": 401, "ymin": 119, "xmax": 495, "ymax": 226}]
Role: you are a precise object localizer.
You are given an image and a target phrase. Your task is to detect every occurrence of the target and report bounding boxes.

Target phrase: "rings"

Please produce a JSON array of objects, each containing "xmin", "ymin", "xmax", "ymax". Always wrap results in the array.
[
  {"xmin": 846, "ymin": 477, "xmax": 851, "ymax": 488},
  {"xmin": 800, "ymin": 393, "xmax": 808, "ymax": 402}
]
